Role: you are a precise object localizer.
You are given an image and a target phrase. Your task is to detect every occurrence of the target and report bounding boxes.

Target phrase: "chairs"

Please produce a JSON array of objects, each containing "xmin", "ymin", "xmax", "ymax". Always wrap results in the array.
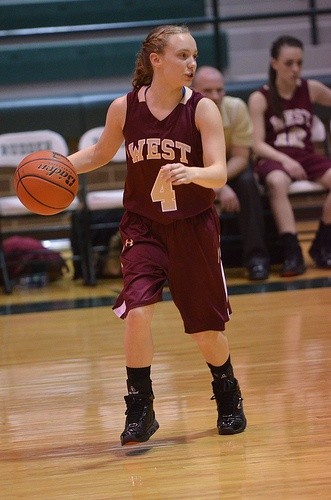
[{"xmin": 0, "ymin": 112, "xmax": 331, "ymax": 296}]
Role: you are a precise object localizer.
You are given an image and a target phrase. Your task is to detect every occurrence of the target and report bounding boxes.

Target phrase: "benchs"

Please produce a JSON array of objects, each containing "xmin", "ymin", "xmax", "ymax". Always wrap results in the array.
[{"xmin": 0, "ymin": 0, "xmax": 230, "ymax": 89}]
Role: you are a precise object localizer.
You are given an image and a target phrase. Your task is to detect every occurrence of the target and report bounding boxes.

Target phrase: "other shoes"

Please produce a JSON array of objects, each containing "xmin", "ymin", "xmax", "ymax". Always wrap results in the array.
[{"xmin": 251, "ymin": 264, "xmax": 268, "ymax": 279}]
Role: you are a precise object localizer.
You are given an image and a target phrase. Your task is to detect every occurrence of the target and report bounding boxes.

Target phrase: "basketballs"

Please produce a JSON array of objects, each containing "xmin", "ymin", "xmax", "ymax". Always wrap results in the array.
[{"xmin": 15, "ymin": 150, "xmax": 78, "ymax": 215}]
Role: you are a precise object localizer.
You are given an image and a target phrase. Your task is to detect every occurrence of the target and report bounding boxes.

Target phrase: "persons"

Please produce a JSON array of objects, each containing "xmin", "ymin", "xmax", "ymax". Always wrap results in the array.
[
  {"xmin": 247, "ymin": 36, "xmax": 331, "ymax": 277},
  {"xmin": 192, "ymin": 66, "xmax": 270, "ymax": 280},
  {"xmin": 66, "ymin": 24, "xmax": 247, "ymax": 447}
]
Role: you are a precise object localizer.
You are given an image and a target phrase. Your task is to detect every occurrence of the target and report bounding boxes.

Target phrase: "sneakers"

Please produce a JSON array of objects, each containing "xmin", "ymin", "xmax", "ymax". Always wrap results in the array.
[
  {"xmin": 309, "ymin": 220, "xmax": 331, "ymax": 268},
  {"xmin": 211, "ymin": 377, "xmax": 246, "ymax": 435},
  {"xmin": 279, "ymin": 233, "xmax": 305, "ymax": 275},
  {"xmin": 120, "ymin": 393, "xmax": 159, "ymax": 446}
]
